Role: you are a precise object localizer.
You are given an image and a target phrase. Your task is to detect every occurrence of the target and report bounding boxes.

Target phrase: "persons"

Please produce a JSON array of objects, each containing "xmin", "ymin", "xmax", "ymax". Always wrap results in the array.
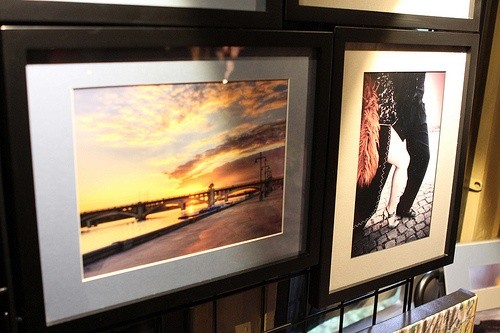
[{"xmin": 351, "ymin": 72, "xmax": 432, "ymax": 232}]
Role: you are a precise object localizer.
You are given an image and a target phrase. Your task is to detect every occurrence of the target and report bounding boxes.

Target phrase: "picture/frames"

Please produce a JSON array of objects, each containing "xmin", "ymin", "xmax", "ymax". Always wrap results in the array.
[
  {"xmin": 306, "ymin": 28, "xmax": 482, "ymax": 307},
  {"xmin": 2, "ymin": 22, "xmax": 325, "ymax": 332},
  {"xmin": 1, "ymin": 1, "xmax": 287, "ymax": 32},
  {"xmin": 283, "ymin": 1, "xmax": 485, "ymax": 34}
]
